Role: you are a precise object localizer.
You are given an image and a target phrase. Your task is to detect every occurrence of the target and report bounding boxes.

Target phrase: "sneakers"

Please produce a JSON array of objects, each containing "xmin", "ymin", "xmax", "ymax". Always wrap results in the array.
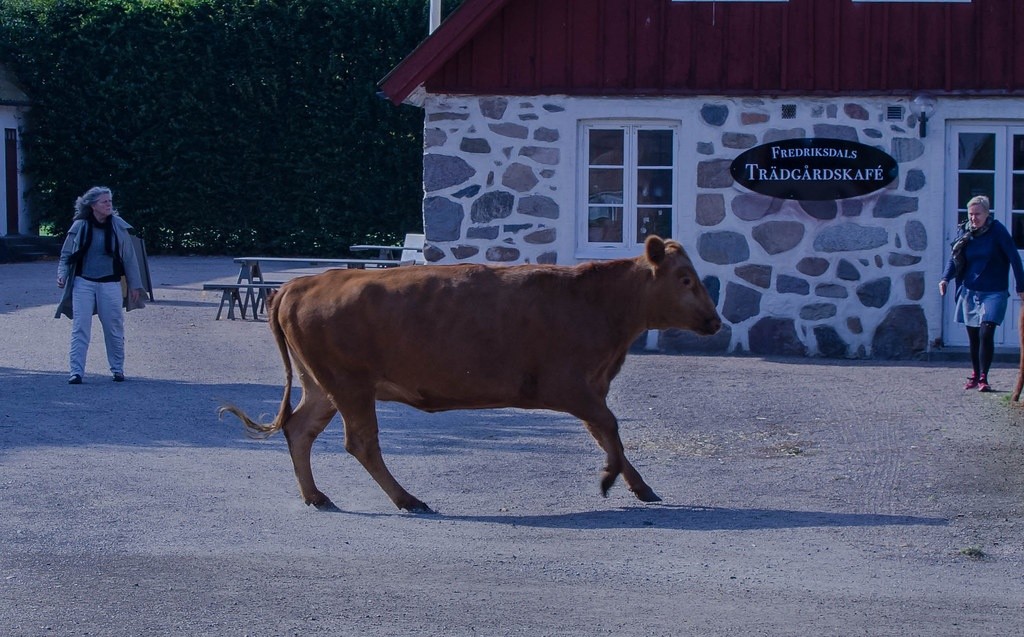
[
  {"xmin": 964, "ymin": 371, "xmax": 978, "ymax": 389},
  {"xmin": 978, "ymin": 374, "xmax": 991, "ymax": 392}
]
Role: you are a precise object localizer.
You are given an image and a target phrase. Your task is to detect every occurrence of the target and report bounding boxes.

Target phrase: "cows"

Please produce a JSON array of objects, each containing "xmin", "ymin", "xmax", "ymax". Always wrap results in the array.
[{"xmin": 215, "ymin": 234, "xmax": 724, "ymax": 516}]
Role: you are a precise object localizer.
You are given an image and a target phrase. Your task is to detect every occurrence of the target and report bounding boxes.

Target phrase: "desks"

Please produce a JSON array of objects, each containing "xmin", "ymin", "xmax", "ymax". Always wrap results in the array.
[
  {"xmin": 232, "ymin": 257, "xmax": 416, "ymax": 322},
  {"xmin": 350, "ymin": 244, "xmax": 423, "ymax": 259}
]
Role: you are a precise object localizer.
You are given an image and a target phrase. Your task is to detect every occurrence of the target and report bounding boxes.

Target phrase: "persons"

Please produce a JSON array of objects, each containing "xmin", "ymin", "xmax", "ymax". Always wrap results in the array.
[
  {"xmin": 54, "ymin": 185, "xmax": 150, "ymax": 384},
  {"xmin": 938, "ymin": 195, "xmax": 1024, "ymax": 393}
]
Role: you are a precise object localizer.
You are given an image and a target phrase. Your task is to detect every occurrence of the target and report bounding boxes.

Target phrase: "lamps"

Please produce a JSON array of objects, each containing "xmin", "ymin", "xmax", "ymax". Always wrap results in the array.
[{"xmin": 910, "ymin": 91, "xmax": 939, "ymax": 139}]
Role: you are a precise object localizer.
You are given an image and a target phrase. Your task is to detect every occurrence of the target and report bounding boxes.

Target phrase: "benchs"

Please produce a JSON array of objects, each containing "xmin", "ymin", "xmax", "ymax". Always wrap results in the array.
[{"xmin": 203, "ymin": 281, "xmax": 288, "ymax": 320}]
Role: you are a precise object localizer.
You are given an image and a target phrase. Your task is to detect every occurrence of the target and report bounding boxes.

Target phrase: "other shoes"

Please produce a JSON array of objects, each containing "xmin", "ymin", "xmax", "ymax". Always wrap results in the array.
[
  {"xmin": 68, "ymin": 374, "xmax": 81, "ymax": 384},
  {"xmin": 113, "ymin": 373, "xmax": 125, "ymax": 382}
]
